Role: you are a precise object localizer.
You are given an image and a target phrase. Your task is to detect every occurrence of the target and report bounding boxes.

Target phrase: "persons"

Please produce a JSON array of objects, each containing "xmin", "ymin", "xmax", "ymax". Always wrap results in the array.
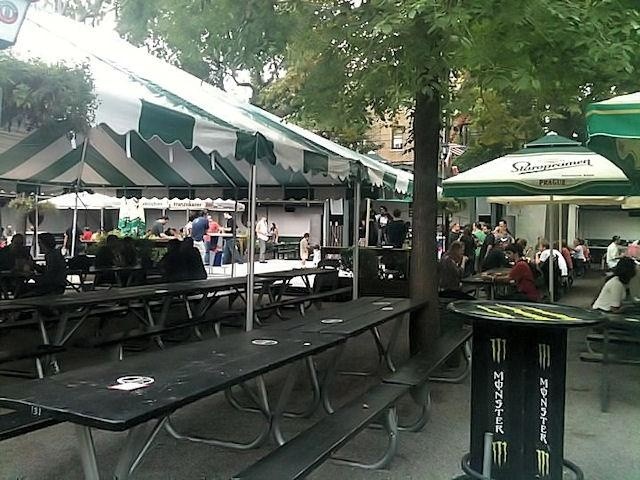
[
  {"xmin": 473, "ymin": 222, "xmax": 486, "ymax": 271},
  {"xmin": 82, "ymin": 227, "xmax": 93, "ymax": 240},
  {"xmin": 218, "ymin": 211, "xmax": 243, "ymax": 264},
  {"xmin": 457, "ymin": 226, "xmax": 477, "ymax": 275},
  {"xmin": 606, "ymin": 235, "xmax": 624, "ymax": 270},
  {"xmin": 95, "ymin": 234, "xmax": 119, "ymax": 283},
  {"xmin": 0, "ymin": 227, "xmax": 7, "ymax": 247},
  {"xmin": 300, "ymin": 233, "xmax": 310, "ymax": 267},
  {"xmin": 387, "ymin": 209, "xmax": 405, "ymax": 248},
  {"xmin": 269, "ymin": 223, "xmax": 278, "ymax": 244},
  {"xmin": 479, "ymin": 223, "xmax": 495, "ymax": 273},
  {"xmin": 448, "ymin": 222, "xmax": 458, "ymax": 245},
  {"xmin": 375, "ymin": 206, "xmax": 387, "ymax": 245},
  {"xmin": 536, "ymin": 241, "xmax": 548, "ymax": 264},
  {"xmin": 555, "ymin": 240, "xmax": 573, "ymax": 270},
  {"xmin": 63, "ymin": 219, "xmax": 84, "ymax": 256},
  {"xmin": 570, "ymin": 238, "xmax": 584, "ymax": 260},
  {"xmin": 4, "ymin": 224, "xmax": 13, "ymax": 240},
  {"xmin": 482, "ymin": 240, "xmax": 509, "ymax": 271},
  {"xmin": 15, "ymin": 232, "xmax": 67, "ymax": 321},
  {"xmin": 151, "ymin": 215, "xmax": 174, "ymax": 239},
  {"xmin": 204, "ymin": 215, "xmax": 221, "ymax": 265},
  {"xmin": 0, "ymin": 233, "xmax": 33, "ymax": 297},
  {"xmin": 579, "ymin": 239, "xmax": 588, "ymax": 258},
  {"xmin": 255, "ymin": 216, "xmax": 269, "ymax": 263},
  {"xmin": 313, "ymin": 244, "xmax": 321, "ymax": 267},
  {"xmin": 183, "ymin": 216, "xmax": 192, "ymax": 237},
  {"xmin": 499, "ymin": 221, "xmax": 515, "ymax": 245},
  {"xmin": 438, "ymin": 240, "xmax": 477, "ymax": 325},
  {"xmin": 494, "ymin": 226, "xmax": 500, "ymax": 241},
  {"xmin": 496, "ymin": 243, "xmax": 537, "ymax": 302},
  {"xmin": 592, "ymin": 257, "xmax": 636, "ymax": 314},
  {"xmin": 192, "ymin": 209, "xmax": 209, "ymax": 268}
]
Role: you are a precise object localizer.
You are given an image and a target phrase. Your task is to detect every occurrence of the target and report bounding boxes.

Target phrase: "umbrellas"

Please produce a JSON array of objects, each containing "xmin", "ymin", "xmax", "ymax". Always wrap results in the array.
[
  {"xmin": 485, "ymin": 195, "xmax": 625, "ymax": 253},
  {"xmin": 441, "ymin": 128, "xmax": 632, "ymax": 302},
  {"xmin": 37, "ymin": 191, "xmax": 121, "ymax": 257}
]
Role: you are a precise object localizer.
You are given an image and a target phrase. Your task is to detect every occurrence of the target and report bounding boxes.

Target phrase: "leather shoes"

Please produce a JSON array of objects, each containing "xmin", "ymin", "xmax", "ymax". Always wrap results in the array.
[
  {"xmin": 260, "ymin": 261, "xmax": 269, "ymax": 264},
  {"xmin": 203, "ymin": 263, "xmax": 210, "ymax": 266}
]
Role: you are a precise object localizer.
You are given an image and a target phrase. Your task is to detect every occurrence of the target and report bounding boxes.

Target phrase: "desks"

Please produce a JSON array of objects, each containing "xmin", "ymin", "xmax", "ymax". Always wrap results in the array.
[
  {"xmin": 274, "ymin": 242, "xmax": 300, "ymax": 259},
  {"xmin": 0, "ymin": 265, "xmax": 158, "ymax": 302},
  {"xmin": 446, "ymin": 299, "xmax": 607, "ymax": 480},
  {"xmin": 0, "ymin": 333, "xmax": 347, "ymax": 480},
  {"xmin": 258, "ymin": 296, "xmax": 430, "ymax": 420},
  {"xmin": 254, "ymin": 269, "xmax": 338, "ymax": 315},
  {"xmin": 111, "ymin": 276, "xmax": 288, "ymax": 351},
  {"xmin": 460, "ymin": 267, "xmax": 513, "ymax": 301},
  {"xmin": 0, "ymin": 288, "xmax": 157, "ymax": 379}
]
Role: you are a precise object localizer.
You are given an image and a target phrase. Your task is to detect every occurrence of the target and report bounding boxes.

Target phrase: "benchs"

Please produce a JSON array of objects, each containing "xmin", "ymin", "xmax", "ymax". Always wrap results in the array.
[
  {"xmin": 234, "ymin": 381, "xmax": 412, "ymax": 480},
  {"xmin": 168, "ymin": 303, "xmax": 287, "ymax": 341},
  {"xmin": 383, "ymin": 325, "xmax": 474, "ymax": 434},
  {"xmin": 439, "ymin": 286, "xmax": 477, "ymax": 303},
  {"xmin": 280, "ymin": 286, "xmax": 352, "ymax": 317},
  {"xmin": 69, "ymin": 324, "xmax": 174, "ymax": 361},
  {"xmin": 0, "ymin": 409, "xmax": 101, "ymax": 480},
  {"xmin": 580, "ymin": 331, "xmax": 640, "ymax": 364},
  {"xmin": 0, "ymin": 345, "xmax": 66, "ymax": 380}
]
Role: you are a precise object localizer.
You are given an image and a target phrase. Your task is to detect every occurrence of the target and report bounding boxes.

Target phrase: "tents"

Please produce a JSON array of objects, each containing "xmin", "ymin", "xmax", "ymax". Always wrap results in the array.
[
  {"xmin": 0, "ymin": 17, "xmax": 257, "ymax": 332},
  {"xmin": 356, "ymin": 154, "xmax": 446, "ymax": 295},
  {"xmin": 585, "ymin": 90, "xmax": 640, "ymax": 196},
  {"xmin": 24, "ymin": 7, "xmax": 357, "ymax": 312}
]
